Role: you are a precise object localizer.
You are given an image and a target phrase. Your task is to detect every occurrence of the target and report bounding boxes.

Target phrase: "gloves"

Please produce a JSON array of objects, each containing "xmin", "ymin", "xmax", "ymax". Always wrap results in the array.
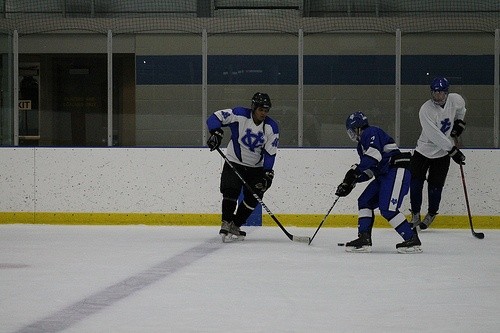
[
  {"xmin": 450, "ymin": 119, "xmax": 467, "ymax": 138},
  {"xmin": 334, "ymin": 181, "xmax": 357, "ymax": 198},
  {"xmin": 254, "ymin": 169, "xmax": 274, "ymax": 194},
  {"xmin": 342, "ymin": 163, "xmax": 360, "ymax": 184},
  {"xmin": 207, "ymin": 128, "xmax": 224, "ymax": 152},
  {"xmin": 448, "ymin": 145, "xmax": 466, "ymax": 165}
]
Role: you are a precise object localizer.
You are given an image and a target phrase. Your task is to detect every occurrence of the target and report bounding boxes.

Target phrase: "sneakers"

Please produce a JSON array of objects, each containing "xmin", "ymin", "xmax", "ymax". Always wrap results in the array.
[
  {"xmin": 226, "ymin": 224, "xmax": 246, "ymax": 241},
  {"xmin": 345, "ymin": 232, "xmax": 373, "ymax": 252},
  {"xmin": 219, "ymin": 220, "xmax": 232, "ymax": 242},
  {"xmin": 419, "ymin": 213, "xmax": 437, "ymax": 231},
  {"xmin": 396, "ymin": 226, "xmax": 423, "ymax": 254},
  {"xmin": 408, "ymin": 212, "xmax": 421, "ymax": 228}
]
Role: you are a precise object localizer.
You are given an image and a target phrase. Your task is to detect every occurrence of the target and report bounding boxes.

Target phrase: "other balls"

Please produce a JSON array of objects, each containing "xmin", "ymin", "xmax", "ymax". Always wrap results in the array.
[{"xmin": 337, "ymin": 243, "xmax": 344, "ymax": 246}]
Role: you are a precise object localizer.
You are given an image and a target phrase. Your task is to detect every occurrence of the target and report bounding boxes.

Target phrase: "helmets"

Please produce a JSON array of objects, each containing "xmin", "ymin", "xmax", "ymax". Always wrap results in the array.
[
  {"xmin": 252, "ymin": 92, "xmax": 272, "ymax": 108},
  {"xmin": 430, "ymin": 77, "xmax": 449, "ymax": 92},
  {"xmin": 345, "ymin": 111, "xmax": 368, "ymax": 130}
]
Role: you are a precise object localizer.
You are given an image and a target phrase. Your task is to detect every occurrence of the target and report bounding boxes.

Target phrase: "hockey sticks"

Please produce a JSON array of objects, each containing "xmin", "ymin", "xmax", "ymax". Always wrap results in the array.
[
  {"xmin": 214, "ymin": 144, "xmax": 310, "ymax": 244},
  {"xmin": 309, "ymin": 197, "xmax": 340, "ymax": 245},
  {"xmin": 455, "ymin": 135, "xmax": 485, "ymax": 239}
]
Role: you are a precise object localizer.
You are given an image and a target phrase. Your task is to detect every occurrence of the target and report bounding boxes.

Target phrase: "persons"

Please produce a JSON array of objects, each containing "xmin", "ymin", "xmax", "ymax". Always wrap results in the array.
[
  {"xmin": 206, "ymin": 92, "xmax": 280, "ymax": 236},
  {"xmin": 334, "ymin": 112, "xmax": 422, "ymax": 249},
  {"xmin": 409, "ymin": 77, "xmax": 467, "ymax": 229}
]
